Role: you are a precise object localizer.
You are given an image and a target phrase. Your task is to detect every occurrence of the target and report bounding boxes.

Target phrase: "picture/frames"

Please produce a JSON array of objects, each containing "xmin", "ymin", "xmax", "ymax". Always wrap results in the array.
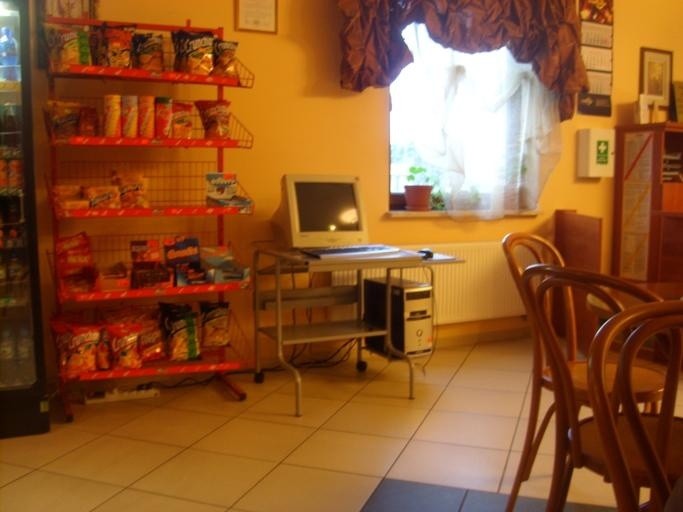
[
  {"xmin": 233, "ymin": 0, "xmax": 277, "ymax": 35},
  {"xmin": 638, "ymin": 46, "xmax": 673, "ymax": 107}
]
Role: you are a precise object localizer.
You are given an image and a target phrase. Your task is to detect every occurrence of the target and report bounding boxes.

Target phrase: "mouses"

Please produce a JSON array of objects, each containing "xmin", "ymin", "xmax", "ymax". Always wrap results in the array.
[{"xmin": 419, "ymin": 248, "xmax": 434, "ymax": 258}]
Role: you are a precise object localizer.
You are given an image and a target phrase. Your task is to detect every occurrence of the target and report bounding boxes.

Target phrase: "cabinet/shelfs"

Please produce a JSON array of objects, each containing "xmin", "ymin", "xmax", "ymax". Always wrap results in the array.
[
  {"xmin": 42, "ymin": 16, "xmax": 256, "ymax": 422},
  {"xmin": 609, "ymin": 124, "xmax": 683, "ymax": 363}
]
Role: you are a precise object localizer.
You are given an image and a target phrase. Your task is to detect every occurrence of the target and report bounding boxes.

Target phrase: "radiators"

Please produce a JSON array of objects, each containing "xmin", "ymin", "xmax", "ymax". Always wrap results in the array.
[{"xmin": 324, "ymin": 241, "xmax": 541, "ymax": 328}]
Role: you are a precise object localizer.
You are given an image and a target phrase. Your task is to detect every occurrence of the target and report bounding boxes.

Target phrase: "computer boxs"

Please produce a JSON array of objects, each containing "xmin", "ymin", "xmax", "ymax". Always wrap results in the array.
[{"xmin": 363, "ymin": 276, "xmax": 433, "ymax": 356}]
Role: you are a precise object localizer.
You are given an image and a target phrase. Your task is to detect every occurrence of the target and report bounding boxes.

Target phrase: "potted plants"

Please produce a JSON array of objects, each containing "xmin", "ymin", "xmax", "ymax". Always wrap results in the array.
[{"xmin": 403, "ymin": 163, "xmax": 433, "ymax": 212}]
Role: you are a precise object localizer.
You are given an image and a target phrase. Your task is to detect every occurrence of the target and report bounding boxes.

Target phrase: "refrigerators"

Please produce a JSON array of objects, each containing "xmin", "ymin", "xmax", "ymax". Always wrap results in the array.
[{"xmin": 0, "ymin": 0, "xmax": 50, "ymax": 440}]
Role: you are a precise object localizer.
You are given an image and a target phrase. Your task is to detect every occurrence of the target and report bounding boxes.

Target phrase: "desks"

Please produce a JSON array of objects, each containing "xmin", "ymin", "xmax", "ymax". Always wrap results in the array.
[{"xmin": 252, "ymin": 248, "xmax": 466, "ymax": 418}]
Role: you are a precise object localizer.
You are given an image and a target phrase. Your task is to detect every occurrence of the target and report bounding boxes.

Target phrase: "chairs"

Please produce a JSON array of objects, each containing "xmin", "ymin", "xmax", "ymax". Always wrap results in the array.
[{"xmin": 500, "ymin": 228, "xmax": 682, "ymax": 510}]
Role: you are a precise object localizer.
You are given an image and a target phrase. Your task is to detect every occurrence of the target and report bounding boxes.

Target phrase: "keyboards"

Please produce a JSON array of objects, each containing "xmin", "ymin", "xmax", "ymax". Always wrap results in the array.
[{"xmin": 298, "ymin": 244, "xmax": 399, "ymax": 260}]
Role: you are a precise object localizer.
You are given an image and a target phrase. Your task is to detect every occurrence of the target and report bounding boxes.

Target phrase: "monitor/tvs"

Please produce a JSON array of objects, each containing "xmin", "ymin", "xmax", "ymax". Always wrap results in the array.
[{"xmin": 270, "ymin": 173, "xmax": 367, "ymax": 250}]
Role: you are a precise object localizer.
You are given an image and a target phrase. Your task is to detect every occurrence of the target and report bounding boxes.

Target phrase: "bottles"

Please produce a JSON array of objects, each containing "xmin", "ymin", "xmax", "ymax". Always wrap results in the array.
[{"xmin": 0, "ymin": 28, "xmax": 36, "ymax": 387}]
